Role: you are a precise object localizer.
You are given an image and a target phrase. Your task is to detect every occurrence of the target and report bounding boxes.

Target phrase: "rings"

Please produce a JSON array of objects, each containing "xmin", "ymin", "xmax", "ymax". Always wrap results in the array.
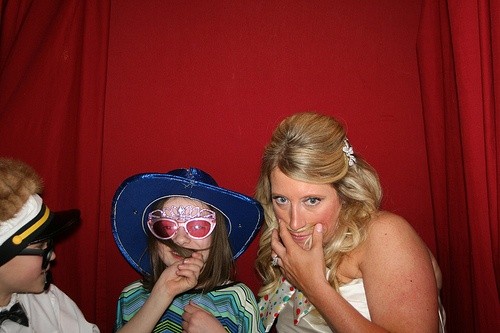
[{"xmin": 271, "ymin": 255, "xmax": 280, "ymax": 267}]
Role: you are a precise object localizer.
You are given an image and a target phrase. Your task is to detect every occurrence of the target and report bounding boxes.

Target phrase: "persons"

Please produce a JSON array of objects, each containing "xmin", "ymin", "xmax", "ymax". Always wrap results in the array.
[
  {"xmin": 255, "ymin": 112, "xmax": 446, "ymax": 333},
  {"xmin": 0, "ymin": 158, "xmax": 204, "ymax": 333},
  {"xmin": 110, "ymin": 168, "xmax": 267, "ymax": 333}
]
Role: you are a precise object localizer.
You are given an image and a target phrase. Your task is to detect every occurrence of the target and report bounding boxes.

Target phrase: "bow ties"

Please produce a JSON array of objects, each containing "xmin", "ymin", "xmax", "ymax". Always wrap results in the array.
[{"xmin": 0, "ymin": 302, "xmax": 29, "ymax": 327}]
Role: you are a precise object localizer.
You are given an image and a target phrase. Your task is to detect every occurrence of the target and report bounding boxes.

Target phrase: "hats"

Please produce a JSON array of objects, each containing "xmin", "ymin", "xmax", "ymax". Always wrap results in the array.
[
  {"xmin": 110, "ymin": 168, "xmax": 265, "ymax": 278},
  {"xmin": 0, "ymin": 192, "xmax": 80, "ymax": 266}
]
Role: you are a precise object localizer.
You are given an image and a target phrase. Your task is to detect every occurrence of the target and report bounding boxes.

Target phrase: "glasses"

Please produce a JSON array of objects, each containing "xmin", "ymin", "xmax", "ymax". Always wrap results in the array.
[
  {"xmin": 146, "ymin": 205, "xmax": 216, "ymax": 240},
  {"xmin": 16, "ymin": 240, "xmax": 55, "ymax": 270}
]
